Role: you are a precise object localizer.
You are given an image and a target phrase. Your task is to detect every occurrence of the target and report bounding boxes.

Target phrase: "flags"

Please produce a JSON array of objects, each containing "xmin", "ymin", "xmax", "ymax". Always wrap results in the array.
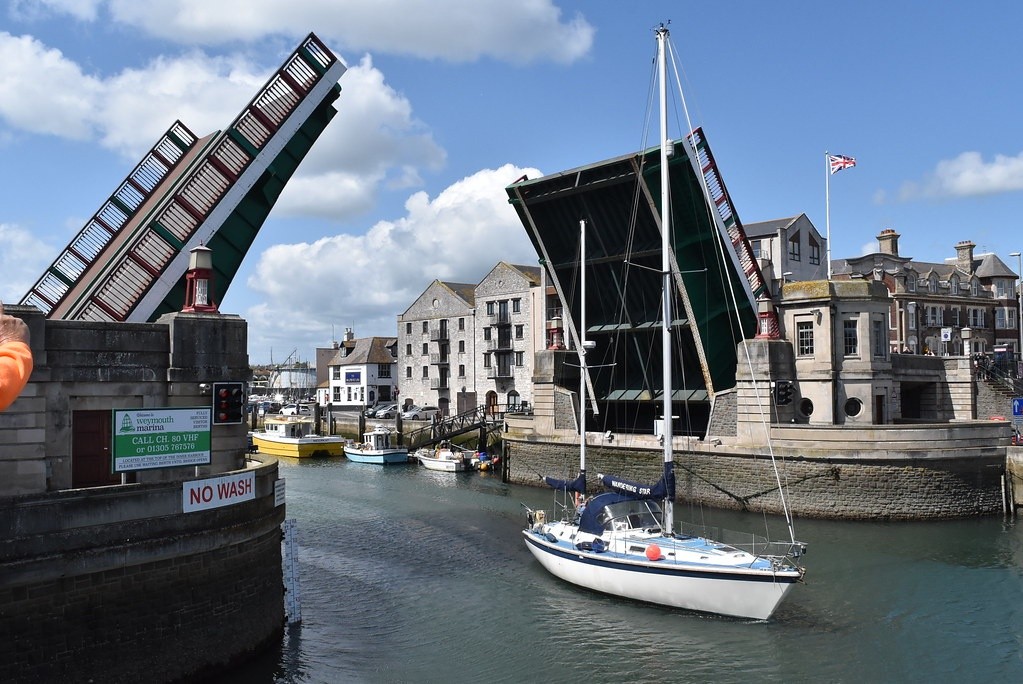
[{"xmin": 829, "ymin": 155, "xmax": 855, "ymax": 174}]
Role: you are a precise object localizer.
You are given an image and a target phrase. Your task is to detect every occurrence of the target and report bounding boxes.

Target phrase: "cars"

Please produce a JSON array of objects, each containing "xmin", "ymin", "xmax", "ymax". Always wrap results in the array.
[
  {"xmin": 374, "ymin": 404, "xmax": 398, "ymax": 419},
  {"xmin": 364, "ymin": 404, "xmax": 388, "ymax": 419},
  {"xmin": 248, "ymin": 400, "xmax": 281, "ymax": 415},
  {"xmin": 278, "ymin": 404, "xmax": 311, "ymax": 417},
  {"xmin": 400, "ymin": 405, "xmax": 442, "ymax": 420}
]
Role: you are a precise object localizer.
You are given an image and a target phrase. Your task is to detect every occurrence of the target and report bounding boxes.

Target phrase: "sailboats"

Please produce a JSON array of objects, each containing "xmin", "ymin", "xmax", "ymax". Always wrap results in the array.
[{"xmin": 520, "ymin": 18, "xmax": 813, "ymax": 623}]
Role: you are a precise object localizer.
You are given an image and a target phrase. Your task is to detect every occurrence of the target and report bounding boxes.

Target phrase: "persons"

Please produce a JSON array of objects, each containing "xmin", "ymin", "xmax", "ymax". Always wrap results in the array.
[
  {"xmin": 970, "ymin": 351, "xmax": 997, "ymax": 383},
  {"xmin": 1015, "ymin": 430, "xmax": 1021, "ymax": 445},
  {"xmin": 892, "ymin": 346, "xmax": 935, "ymax": 356}
]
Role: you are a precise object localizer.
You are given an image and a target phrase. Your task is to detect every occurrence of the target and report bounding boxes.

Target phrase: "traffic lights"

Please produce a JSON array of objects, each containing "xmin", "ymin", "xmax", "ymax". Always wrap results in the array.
[{"xmin": 212, "ymin": 381, "xmax": 244, "ymax": 426}]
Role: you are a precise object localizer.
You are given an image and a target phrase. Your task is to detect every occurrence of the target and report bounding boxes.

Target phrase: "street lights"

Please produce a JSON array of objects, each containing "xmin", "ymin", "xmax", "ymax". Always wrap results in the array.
[
  {"xmin": 783, "ymin": 271, "xmax": 794, "ymax": 284},
  {"xmin": 1007, "ymin": 252, "xmax": 1023, "ymax": 355}
]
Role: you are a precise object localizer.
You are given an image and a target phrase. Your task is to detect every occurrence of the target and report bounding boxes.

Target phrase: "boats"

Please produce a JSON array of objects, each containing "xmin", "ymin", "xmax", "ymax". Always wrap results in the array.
[
  {"xmin": 413, "ymin": 439, "xmax": 501, "ymax": 472},
  {"xmin": 249, "ymin": 419, "xmax": 346, "ymax": 459},
  {"xmin": 341, "ymin": 426, "xmax": 410, "ymax": 464}
]
